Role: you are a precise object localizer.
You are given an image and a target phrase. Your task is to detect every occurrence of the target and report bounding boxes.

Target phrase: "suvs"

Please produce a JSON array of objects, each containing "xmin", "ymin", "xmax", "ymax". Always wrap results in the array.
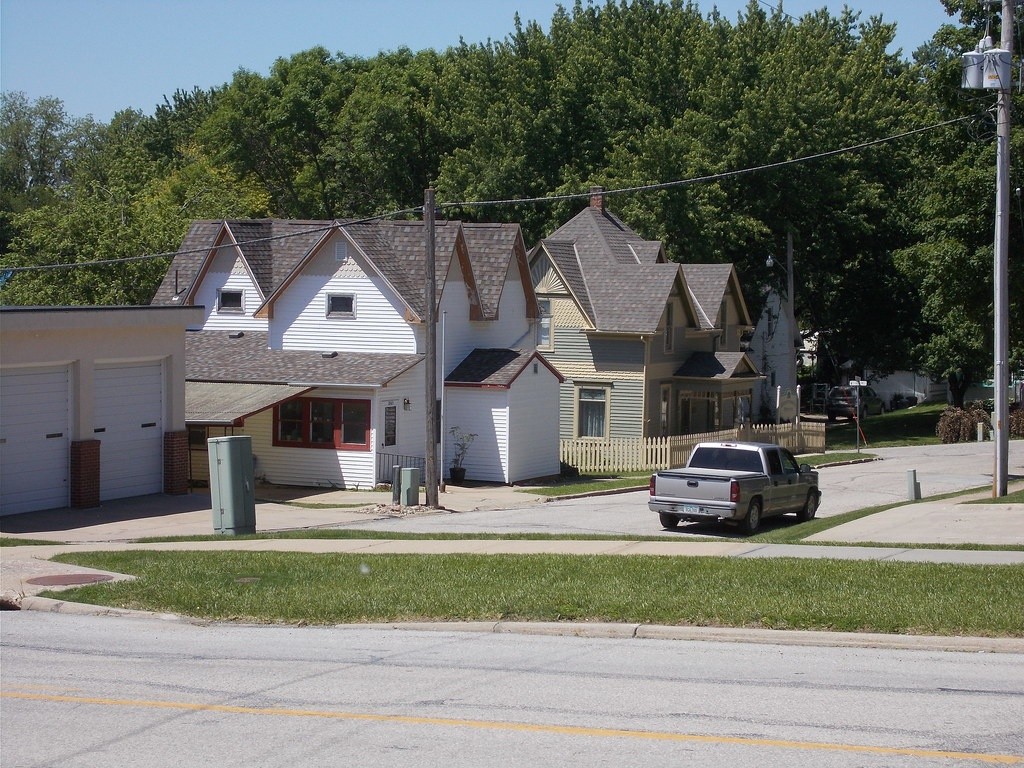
[{"xmin": 827, "ymin": 387, "xmax": 884, "ymax": 423}]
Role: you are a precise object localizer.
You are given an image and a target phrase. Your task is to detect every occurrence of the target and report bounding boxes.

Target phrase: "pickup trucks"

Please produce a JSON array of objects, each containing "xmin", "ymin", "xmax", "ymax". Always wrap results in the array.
[{"xmin": 648, "ymin": 441, "xmax": 822, "ymax": 535}]
